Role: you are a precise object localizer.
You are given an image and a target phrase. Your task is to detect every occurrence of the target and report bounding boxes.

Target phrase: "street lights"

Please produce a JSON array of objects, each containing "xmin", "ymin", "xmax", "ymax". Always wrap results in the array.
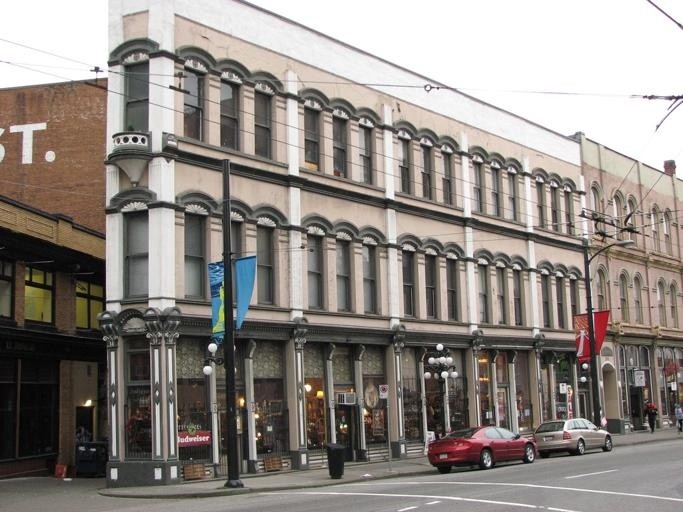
[
  {"xmin": 420, "ymin": 342, "xmax": 457, "ymax": 437},
  {"xmin": 579, "ymin": 237, "xmax": 634, "ymax": 429},
  {"xmin": 579, "ymin": 361, "xmax": 593, "ymax": 422}
]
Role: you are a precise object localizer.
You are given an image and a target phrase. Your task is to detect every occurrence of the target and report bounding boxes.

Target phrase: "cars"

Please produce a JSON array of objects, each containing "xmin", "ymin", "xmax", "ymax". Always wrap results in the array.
[
  {"xmin": 536, "ymin": 415, "xmax": 613, "ymax": 459},
  {"xmin": 426, "ymin": 424, "xmax": 537, "ymax": 475}
]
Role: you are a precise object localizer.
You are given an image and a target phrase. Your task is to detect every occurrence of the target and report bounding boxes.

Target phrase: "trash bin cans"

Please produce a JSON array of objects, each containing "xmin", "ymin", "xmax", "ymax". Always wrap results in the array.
[{"xmin": 325, "ymin": 443, "xmax": 346, "ymax": 479}]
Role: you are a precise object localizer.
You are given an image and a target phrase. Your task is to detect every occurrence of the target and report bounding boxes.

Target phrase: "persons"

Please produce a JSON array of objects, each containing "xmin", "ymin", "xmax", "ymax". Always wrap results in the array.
[
  {"xmin": 673, "ymin": 403, "xmax": 682, "ymax": 433},
  {"xmin": 642, "ymin": 401, "xmax": 658, "ymax": 434},
  {"xmin": 590, "ymin": 406, "xmax": 607, "ymax": 428}
]
